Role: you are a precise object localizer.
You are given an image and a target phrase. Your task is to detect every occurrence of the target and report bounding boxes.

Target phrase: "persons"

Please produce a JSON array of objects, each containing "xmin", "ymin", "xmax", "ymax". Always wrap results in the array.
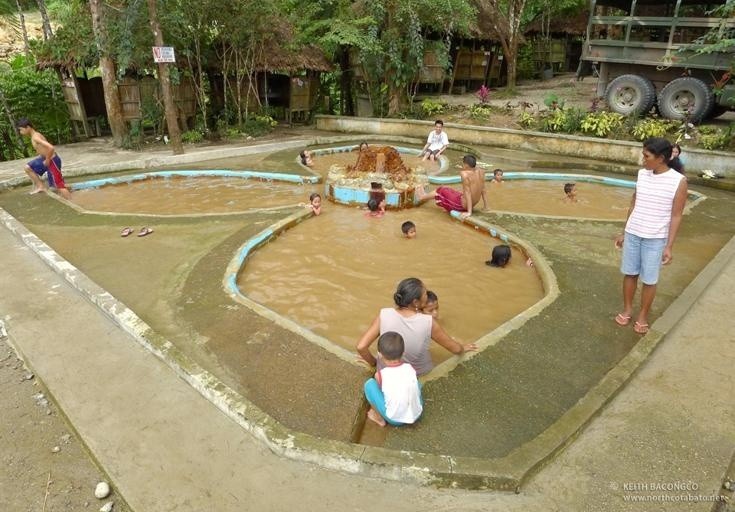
[
  {"xmin": 415, "ymin": 153, "xmax": 490, "ymax": 222},
  {"xmin": 486, "ymin": 245, "xmax": 534, "ymax": 273},
  {"xmin": 612, "ymin": 136, "xmax": 688, "ymax": 334},
  {"xmin": 297, "ymin": 193, "xmax": 324, "ymax": 217},
  {"xmin": 296, "ymin": 141, "xmax": 369, "ymax": 170},
  {"xmin": 417, "ymin": 119, "xmax": 450, "ymax": 163},
  {"xmin": 354, "ymin": 275, "xmax": 480, "ymax": 428},
  {"xmin": 400, "ymin": 219, "xmax": 419, "ymax": 240},
  {"xmin": 16, "ymin": 120, "xmax": 69, "ymax": 198},
  {"xmin": 364, "ymin": 198, "xmax": 387, "ymax": 219},
  {"xmin": 491, "ymin": 169, "xmax": 505, "ymax": 185},
  {"xmin": 562, "ymin": 183, "xmax": 578, "ymax": 204},
  {"xmin": 667, "ymin": 143, "xmax": 682, "ymax": 167}
]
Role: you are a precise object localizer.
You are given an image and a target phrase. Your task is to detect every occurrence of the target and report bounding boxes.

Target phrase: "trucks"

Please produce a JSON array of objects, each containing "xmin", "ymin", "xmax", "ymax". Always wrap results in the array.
[{"xmin": 576, "ymin": 0, "xmax": 734, "ymax": 127}]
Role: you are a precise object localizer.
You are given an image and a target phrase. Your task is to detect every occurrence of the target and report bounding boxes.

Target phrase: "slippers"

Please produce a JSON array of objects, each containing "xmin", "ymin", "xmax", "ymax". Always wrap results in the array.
[
  {"xmin": 634, "ymin": 321, "xmax": 648, "ymax": 334},
  {"xmin": 615, "ymin": 313, "xmax": 631, "ymax": 326}
]
